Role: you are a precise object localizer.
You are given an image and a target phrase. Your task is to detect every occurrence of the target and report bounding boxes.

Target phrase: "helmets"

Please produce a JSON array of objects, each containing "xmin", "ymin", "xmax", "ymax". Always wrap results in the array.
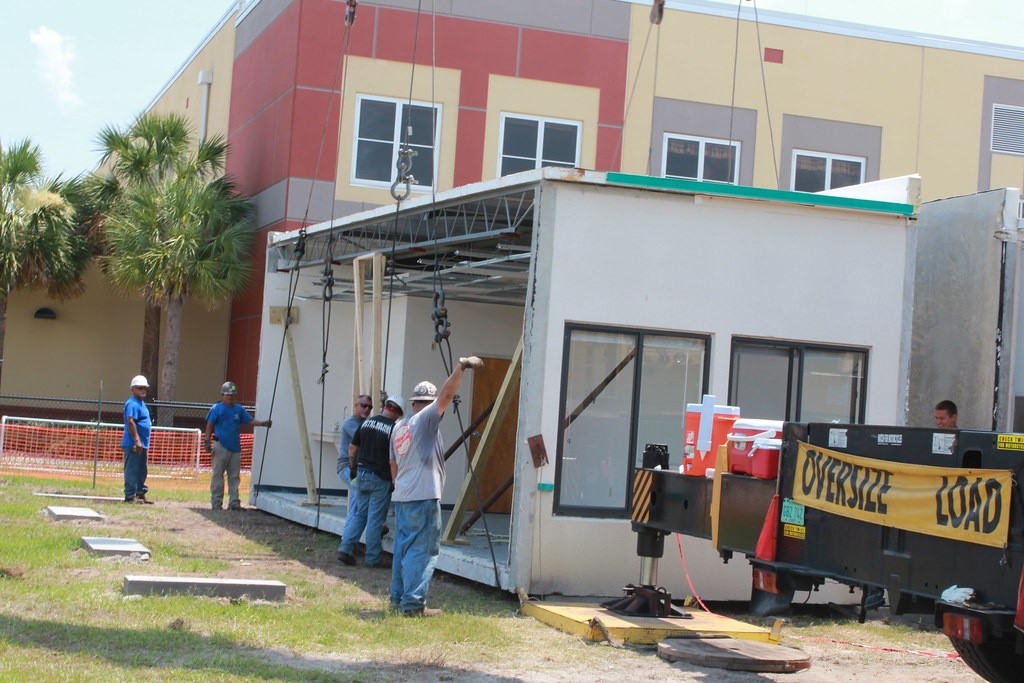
[
  {"xmin": 385, "ymin": 395, "xmax": 406, "ymax": 419},
  {"xmin": 408, "ymin": 381, "xmax": 437, "ymax": 400},
  {"xmin": 130, "ymin": 375, "xmax": 150, "ymax": 388},
  {"xmin": 221, "ymin": 381, "xmax": 238, "ymax": 395}
]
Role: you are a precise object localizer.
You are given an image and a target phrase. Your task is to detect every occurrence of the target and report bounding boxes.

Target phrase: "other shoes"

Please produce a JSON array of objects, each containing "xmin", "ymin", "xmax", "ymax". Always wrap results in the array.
[
  {"xmin": 227, "ymin": 506, "xmax": 247, "ymax": 512},
  {"xmin": 368, "ymin": 559, "xmax": 392, "ymax": 570},
  {"xmin": 337, "ymin": 552, "xmax": 356, "ymax": 566},
  {"xmin": 382, "ymin": 524, "xmax": 389, "ymax": 535},
  {"xmin": 125, "ymin": 497, "xmax": 144, "ymax": 504},
  {"xmin": 212, "ymin": 507, "xmax": 226, "ymax": 514},
  {"xmin": 136, "ymin": 494, "xmax": 154, "ymax": 504}
]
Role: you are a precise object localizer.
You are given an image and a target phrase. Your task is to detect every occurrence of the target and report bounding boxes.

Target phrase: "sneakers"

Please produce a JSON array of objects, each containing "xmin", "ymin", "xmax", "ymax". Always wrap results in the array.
[
  {"xmin": 389, "ymin": 602, "xmax": 402, "ymax": 616},
  {"xmin": 404, "ymin": 605, "xmax": 442, "ymax": 618}
]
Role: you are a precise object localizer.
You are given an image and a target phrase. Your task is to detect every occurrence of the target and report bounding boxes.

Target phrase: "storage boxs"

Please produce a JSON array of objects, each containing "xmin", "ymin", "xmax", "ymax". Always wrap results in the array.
[
  {"xmin": 726, "ymin": 417, "xmax": 784, "ymax": 480},
  {"xmin": 683, "ymin": 394, "xmax": 740, "ymax": 476}
]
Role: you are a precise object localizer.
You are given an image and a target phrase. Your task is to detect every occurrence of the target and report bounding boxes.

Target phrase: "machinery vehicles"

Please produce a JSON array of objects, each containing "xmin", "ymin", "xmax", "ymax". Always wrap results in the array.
[{"xmin": 600, "ymin": 417, "xmax": 1024, "ymax": 683}]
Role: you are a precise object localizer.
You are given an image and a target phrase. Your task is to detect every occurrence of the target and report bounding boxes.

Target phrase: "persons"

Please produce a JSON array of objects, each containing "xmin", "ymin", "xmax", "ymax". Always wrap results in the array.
[
  {"xmin": 336, "ymin": 395, "xmax": 389, "ymax": 557},
  {"xmin": 389, "ymin": 356, "xmax": 483, "ymax": 617},
  {"xmin": 935, "ymin": 400, "xmax": 958, "ymax": 429},
  {"xmin": 121, "ymin": 375, "xmax": 154, "ymax": 504},
  {"xmin": 336, "ymin": 395, "xmax": 406, "ymax": 569},
  {"xmin": 205, "ymin": 382, "xmax": 272, "ymax": 511}
]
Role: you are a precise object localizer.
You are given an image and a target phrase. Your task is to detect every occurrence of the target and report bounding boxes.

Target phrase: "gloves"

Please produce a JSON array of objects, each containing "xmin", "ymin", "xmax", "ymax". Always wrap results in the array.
[
  {"xmin": 263, "ymin": 419, "xmax": 272, "ymax": 428},
  {"xmin": 459, "ymin": 356, "xmax": 484, "ymax": 370},
  {"xmin": 133, "ymin": 445, "xmax": 143, "ymax": 455},
  {"xmin": 350, "ymin": 466, "xmax": 357, "ymax": 481},
  {"xmin": 204, "ymin": 440, "xmax": 211, "ymax": 453},
  {"xmin": 391, "ymin": 481, "xmax": 395, "ymax": 492}
]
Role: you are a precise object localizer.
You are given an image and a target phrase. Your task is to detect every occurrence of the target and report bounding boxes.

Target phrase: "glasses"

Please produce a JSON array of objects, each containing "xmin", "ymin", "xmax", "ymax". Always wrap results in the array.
[
  {"xmin": 361, "ymin": 402, "xmax": 373, "ymax": 410},
  {"xmin": 138, "ymin": 387, "xmax": 148, "ymax": 390}
]
